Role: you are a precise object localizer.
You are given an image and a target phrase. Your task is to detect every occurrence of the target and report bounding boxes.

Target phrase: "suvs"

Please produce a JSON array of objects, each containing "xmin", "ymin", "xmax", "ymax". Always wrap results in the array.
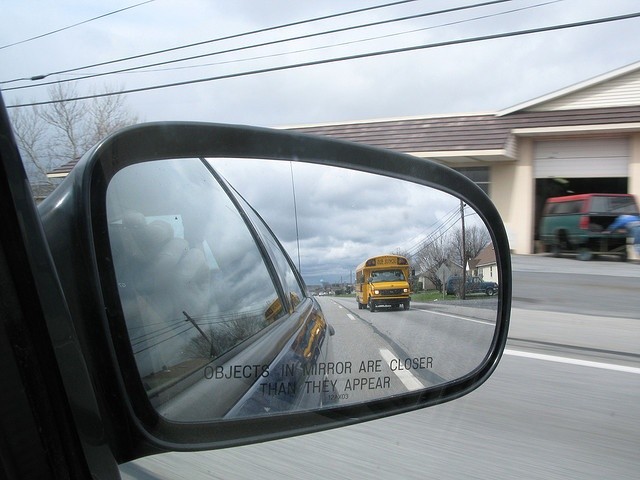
[{"xmin": 445, "ymin": 275, "xmax": 498, "ymax": 299}]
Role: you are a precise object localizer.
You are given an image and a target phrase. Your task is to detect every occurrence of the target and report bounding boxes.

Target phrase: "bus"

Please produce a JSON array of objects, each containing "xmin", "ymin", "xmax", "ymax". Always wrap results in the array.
[{"xmin": 355, "ymin": 255, "xmax": 411, "ymax": 311}]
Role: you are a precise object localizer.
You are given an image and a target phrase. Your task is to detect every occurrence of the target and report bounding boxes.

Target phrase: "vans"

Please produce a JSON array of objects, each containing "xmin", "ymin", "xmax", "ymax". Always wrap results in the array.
[{"xmin": 539, "ymin": 192, "xmax": 640, "ymax": 260}]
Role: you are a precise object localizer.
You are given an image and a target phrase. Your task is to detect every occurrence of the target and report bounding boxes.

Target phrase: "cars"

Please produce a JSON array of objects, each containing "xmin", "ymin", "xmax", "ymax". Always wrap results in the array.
[
  {"xmin": 1, "ymin": 94, "xmax": 515, "ymax": 479},
  {"xmin": 102, "ymin": 158, "xmax": 337, "ymax": 424}
]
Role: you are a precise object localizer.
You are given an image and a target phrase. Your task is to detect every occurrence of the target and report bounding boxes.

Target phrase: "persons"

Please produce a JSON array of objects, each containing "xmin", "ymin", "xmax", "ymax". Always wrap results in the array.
[
  {"xmin": 598, "ymin": 214, "xmax": 639, "ymax": 261},
  {"xmin": 121, "ymin": 206, "xmax": 211, "ymax": 323}
]
